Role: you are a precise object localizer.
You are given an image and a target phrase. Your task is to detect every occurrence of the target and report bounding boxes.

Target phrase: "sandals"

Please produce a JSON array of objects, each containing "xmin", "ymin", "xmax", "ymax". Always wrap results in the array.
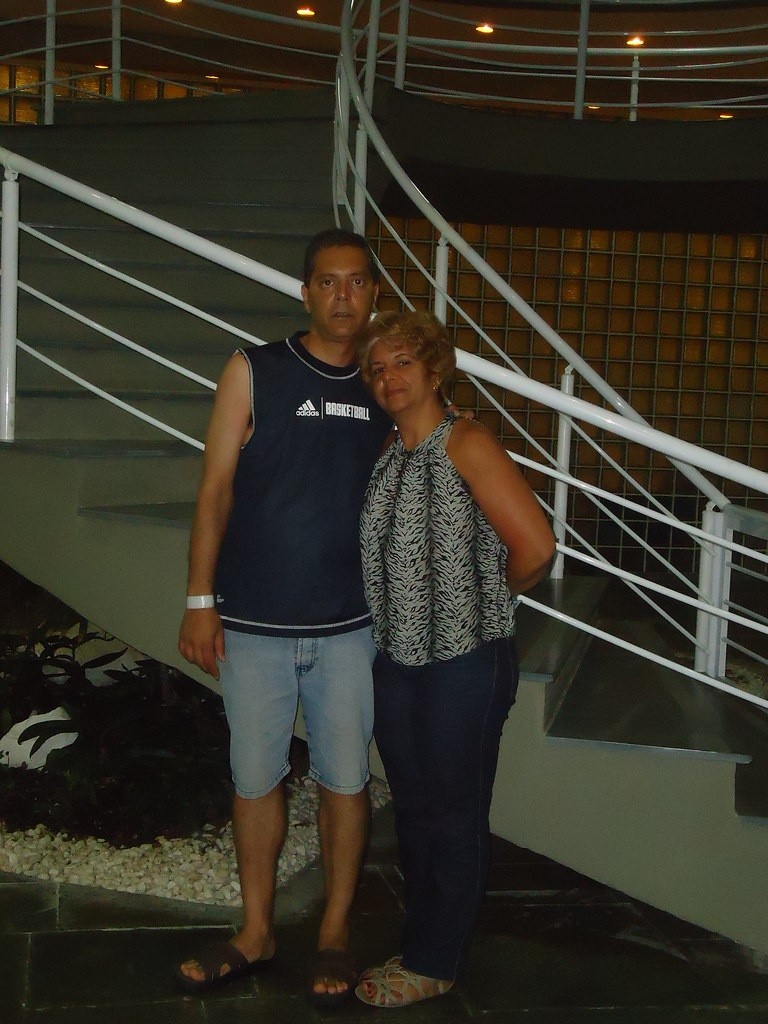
[
  {"xmin": 358, "ymin": 956, "xmax": 404, "ymax": 979},
  {"xmin": 355, "ymin": 967, "xmax": 455, "ymax": 1008}
]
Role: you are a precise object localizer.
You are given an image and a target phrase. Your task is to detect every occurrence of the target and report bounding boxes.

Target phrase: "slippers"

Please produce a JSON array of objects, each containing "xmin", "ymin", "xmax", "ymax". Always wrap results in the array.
[
  {"xmin": 174, "ymin": 941, "xmax": 279, "ymax": 992},
  {"xmin": 307, "ymin": 948, "xmax": 356, "ymax": 1005}
]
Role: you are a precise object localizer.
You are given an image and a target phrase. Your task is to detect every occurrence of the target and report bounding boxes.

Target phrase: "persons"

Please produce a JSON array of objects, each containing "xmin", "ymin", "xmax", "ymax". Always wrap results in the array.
[
  {"xmin": 355, "ymin": 310, "xmax": 558, "ymax": 1011},
  {"xmin": 177, "ymin": 231, "xmax": 475, "ymax": 1006}
]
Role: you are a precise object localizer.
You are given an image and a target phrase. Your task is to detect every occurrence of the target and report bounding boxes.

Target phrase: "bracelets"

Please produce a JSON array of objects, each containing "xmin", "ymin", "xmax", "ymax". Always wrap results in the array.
[{"xmin": 185, "ymin": 593, "xmax": 214, "ymax": 609}]
